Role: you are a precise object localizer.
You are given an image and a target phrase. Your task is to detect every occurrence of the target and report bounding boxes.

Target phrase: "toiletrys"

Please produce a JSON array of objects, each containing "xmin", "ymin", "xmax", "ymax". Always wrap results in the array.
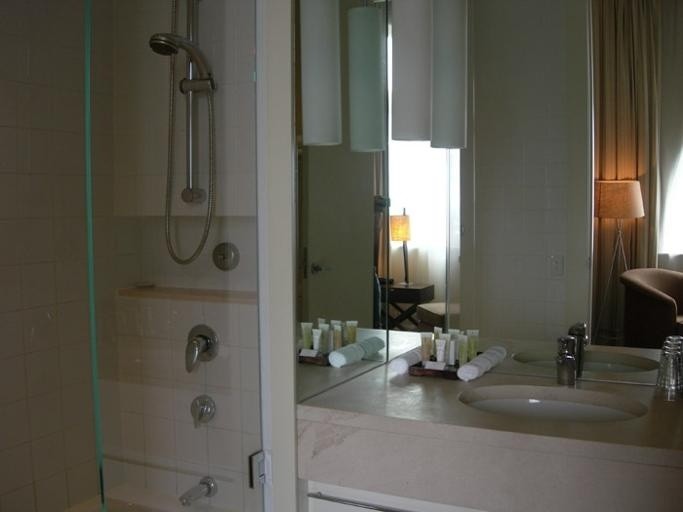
[
  {"xmin": 420, "ymin": 326, "xmax": 480, "ymax": 371},
  {"xmin": 300, "ymin": 317, "xmax": 360, "ymax": 355}
]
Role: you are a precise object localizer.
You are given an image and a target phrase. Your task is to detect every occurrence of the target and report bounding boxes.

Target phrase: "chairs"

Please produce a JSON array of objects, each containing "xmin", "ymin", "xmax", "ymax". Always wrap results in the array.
[{"xmin": 618, "ymin": 267, "xmax": 683, "ymax": 349}]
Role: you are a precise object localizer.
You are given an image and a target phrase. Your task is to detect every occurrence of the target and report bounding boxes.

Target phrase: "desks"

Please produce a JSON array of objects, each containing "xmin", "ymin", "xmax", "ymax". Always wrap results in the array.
[{"xmin": 387, "ymin": 282, "xmax": 434, "ymax": 331}]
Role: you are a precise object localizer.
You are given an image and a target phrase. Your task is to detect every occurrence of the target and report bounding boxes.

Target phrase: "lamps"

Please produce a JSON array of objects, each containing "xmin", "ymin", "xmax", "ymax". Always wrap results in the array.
[
  {"xmin": 299, "ymin": 0, "xmax": 344, "ymax": 148},
  {"xmin": 429, "ymin": 0, "xmax": 469, "ymax": 150},
  {"xmin": 389, "ymin": 0, "xmax": 433, "ymax": 141},
  {"xmin": 347, "ymin": 0, "xmax": 389, "ymax": 154},
  {"xmin": 390, "ymin": 207, "xmax": 412, "ymax": 284},
  {"xmin": 593, "ymin": 180, "xmax": 646, "ymax": 345}
]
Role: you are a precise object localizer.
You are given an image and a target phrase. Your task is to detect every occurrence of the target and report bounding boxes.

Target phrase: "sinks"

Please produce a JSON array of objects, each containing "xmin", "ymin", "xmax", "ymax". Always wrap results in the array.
[
  {"xmin": 512, "ymin": 347, "xmax": 659, "ymax": 373},
  {"xmin": 458, "ymin": 385, "xmax": 649, "ymax": 423}
]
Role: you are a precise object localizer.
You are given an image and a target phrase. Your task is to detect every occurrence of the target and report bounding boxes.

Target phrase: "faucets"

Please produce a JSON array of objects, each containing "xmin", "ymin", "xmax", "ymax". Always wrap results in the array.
[
  {"xmin": 568, "ymin": 321, "xmax": 592, "ymax": 378},
  {"xmin": 554, "ymin": 336, "xmax": 577, "ymax": 387}
]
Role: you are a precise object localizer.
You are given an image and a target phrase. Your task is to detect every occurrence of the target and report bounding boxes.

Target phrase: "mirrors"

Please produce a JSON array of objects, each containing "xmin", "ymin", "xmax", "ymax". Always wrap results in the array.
[
  {"xmin": 446, "ymin": 0, "xmax": 682, "ymax": 387},
  {"xmin": 295, "ymin": 0, "xmax": 449, "ymax": 405}
]
